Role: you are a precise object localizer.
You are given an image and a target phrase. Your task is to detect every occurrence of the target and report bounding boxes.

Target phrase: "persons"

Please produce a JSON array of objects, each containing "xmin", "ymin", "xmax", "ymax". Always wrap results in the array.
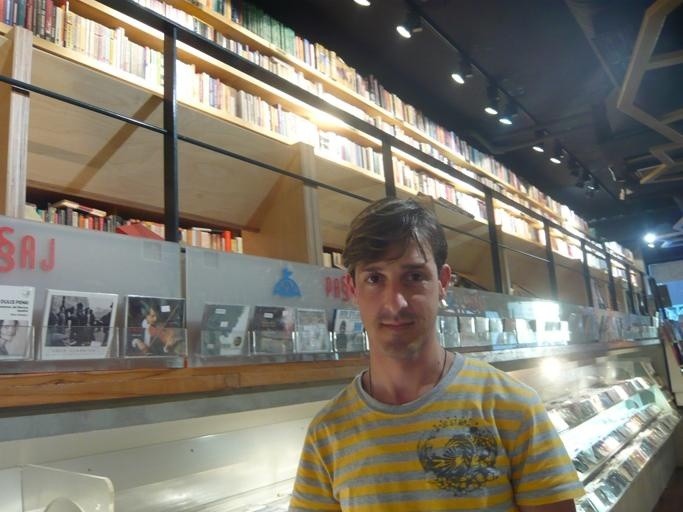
[{"xmin": 287, "ymin": 195, "xmax": 585, "ymax": 511}]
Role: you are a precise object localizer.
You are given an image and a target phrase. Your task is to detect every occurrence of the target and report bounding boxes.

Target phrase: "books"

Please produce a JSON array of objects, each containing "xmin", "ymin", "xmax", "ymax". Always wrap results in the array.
[{"xmin": 1, "ymin": 2, "xmax": 636, "ymax": 362}]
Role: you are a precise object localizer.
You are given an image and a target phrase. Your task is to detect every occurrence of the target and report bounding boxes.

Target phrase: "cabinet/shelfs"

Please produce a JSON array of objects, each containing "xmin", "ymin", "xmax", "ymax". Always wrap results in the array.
[
  {"xmin": 546, "ymin": 364, "xmax": 683, "ymax": 512},
  {"xmin": 0, "ymin": 0, "xmax": 650, "ymax": 408}
]
